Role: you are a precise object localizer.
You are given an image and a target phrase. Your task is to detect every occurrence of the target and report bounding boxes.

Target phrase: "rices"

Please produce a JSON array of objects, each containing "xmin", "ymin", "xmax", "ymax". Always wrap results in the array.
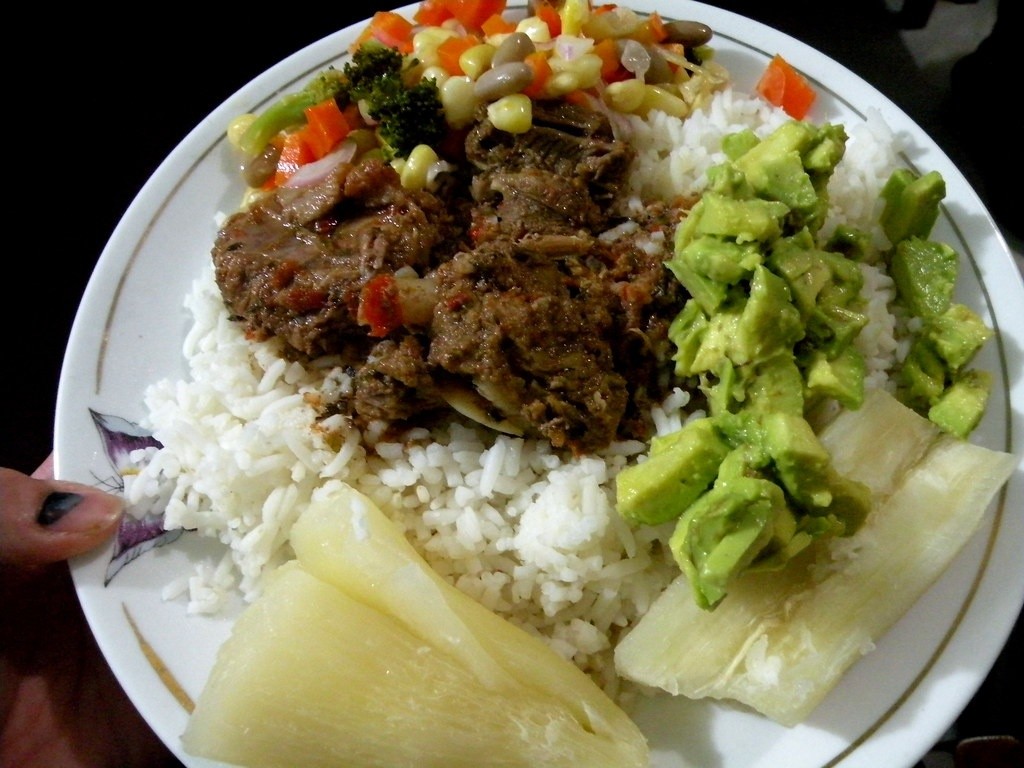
[{"xmin": 124, "ymin": 91, "xmax": 908, "ymax": 692}]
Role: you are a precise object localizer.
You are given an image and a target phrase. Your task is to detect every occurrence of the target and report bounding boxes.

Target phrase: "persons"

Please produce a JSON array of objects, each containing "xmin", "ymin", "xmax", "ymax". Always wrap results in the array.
[{"xmin": 2, "ymin": 453, "xmax": 180, "ymax": 766}]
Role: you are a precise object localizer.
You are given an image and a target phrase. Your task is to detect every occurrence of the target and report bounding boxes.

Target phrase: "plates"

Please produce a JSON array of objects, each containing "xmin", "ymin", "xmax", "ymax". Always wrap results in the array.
[{"xmin": 49, "ymin": 2, "xmax": 1024, "ymax": 768}]
